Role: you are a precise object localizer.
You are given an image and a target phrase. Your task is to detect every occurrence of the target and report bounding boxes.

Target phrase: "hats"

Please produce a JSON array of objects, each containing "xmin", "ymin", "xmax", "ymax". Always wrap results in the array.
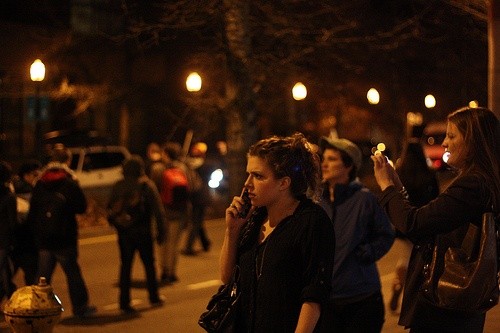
[{"xmin": 318, "ymin": 136, "xmax": 361, "ymax": 170}]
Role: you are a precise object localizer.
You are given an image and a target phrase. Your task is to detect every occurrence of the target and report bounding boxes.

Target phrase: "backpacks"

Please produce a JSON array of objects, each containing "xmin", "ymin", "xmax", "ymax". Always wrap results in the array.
[
  {"xmin": 106, "ymin": 181, "xmax": 150, "ymax": 228},
  {"xmin": 160, "ymin": 168, "xmax": 190, "ymax": 209},
  {"xmin": 27, "ymin": 190, "xmax": 67, "ymax": 242}
]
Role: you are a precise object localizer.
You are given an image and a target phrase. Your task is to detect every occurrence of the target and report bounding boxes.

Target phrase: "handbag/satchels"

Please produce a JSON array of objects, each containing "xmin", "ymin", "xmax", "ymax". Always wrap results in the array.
[
  {"xmin": 197, "ymin": 282, "xmax": 241, "ymax": 333},
  {"xmin": 422, "ymin": 210, "xmax": 500, "ymax": 313}
]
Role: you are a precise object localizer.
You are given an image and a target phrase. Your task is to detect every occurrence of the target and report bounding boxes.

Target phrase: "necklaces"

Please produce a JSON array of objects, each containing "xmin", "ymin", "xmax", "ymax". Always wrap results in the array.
[{"xmin": 255, "ymin": 236, "xmax": 269, "ymax": 280}]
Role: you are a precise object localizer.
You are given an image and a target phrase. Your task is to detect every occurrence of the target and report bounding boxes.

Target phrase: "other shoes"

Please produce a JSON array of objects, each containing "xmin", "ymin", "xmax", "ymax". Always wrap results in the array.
[
  {"xmin": 74, "ymin": 304, "xmax": 97, "ymax": 315},
  {"xmin": 389, "ymin": 282, "xmax": 402, "ymax": 309},
  {"xmin": 150, "ymin": 294, "xmax": 165, "ymax": 305},
  {"xmin": 119, "ymin": 303, "xmax": 134, "ymax": 311}
]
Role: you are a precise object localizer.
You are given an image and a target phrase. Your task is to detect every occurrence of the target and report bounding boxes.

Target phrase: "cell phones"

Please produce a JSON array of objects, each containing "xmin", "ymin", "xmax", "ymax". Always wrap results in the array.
[{"xmin": 236, "ymin": 187, "xmax": 252, "ymax": 219}]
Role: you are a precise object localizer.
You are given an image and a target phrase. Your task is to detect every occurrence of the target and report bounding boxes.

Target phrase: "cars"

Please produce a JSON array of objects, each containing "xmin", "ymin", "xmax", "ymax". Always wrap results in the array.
[{"xmin": 69, "ymin": 145, "xmax": 133, "ymax": 190}]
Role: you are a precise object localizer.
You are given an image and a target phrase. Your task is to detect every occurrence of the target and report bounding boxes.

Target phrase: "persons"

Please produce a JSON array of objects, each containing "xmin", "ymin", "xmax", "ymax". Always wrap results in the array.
[
  {"xmin": 106, "ymin": 141, "xmax": 212, "ymax": 315},
  {"xmin": 0, "ymin": 143, "xmax": 98, "ymax": 317},
  {"xmin": 370, "ymin": 106, "xmax": 500, "ymax": 333},
  {"xmin": 218, "ymin": 130, "xmax": 396, "ymax": 333}
]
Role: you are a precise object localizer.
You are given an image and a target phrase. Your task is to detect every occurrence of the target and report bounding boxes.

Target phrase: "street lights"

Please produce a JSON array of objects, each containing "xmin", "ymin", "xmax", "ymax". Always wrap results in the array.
[
  {"xmin": 366, "ymin": 86, "xmax": 380, "ymax": 144},
  {"xmin": 29, "ymin": 57, "xmax": 45, "ymax": 176},
  {"xmin": 186, "ymin": 71, "xmax": 203, "ymax": 144},
  {"xmin": 292, "ymin": 81, "xmax": 307, "ymax": 135}
]
